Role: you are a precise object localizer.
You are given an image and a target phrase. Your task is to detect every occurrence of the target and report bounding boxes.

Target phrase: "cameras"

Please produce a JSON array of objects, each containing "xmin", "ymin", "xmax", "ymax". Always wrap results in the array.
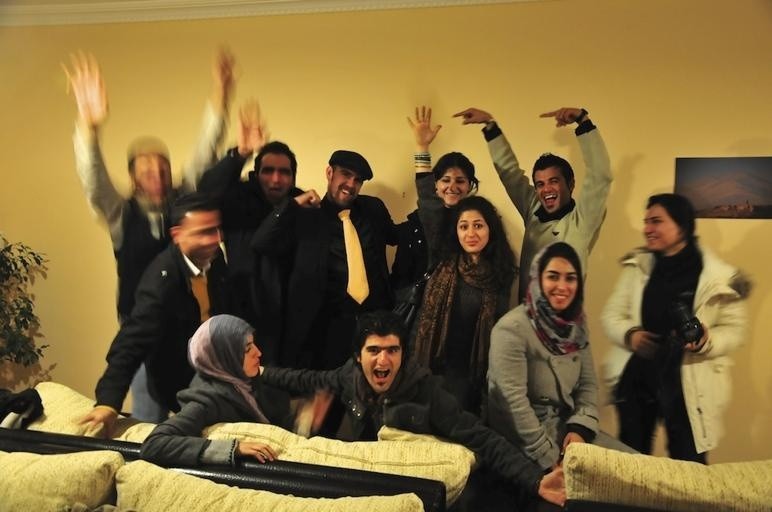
[{"xmin": 669, "ymin": 294, "xmax": 704, "ymax": 342}]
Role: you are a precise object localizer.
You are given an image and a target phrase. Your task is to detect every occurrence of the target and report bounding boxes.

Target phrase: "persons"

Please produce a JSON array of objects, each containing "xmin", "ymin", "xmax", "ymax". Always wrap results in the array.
[
  {"xmin": 482, "ymin": 240, "xmax": 647, "ymax": 471},
  {"xmin": 451, "ymin": 105, "xmax": 616, "ymax": 301},
  {"xmin": 59, "ymin": 40, "xmax": 238, "ymax": 427},
  {"xmin": 597, "ymin": 191, "xmax": 756, "ymax": 467},
  {"xmin": 245, "ymin": 149, "xmax": 412, "ymax": 368},
  {"xmin": 390, "ymin": 149, "xmax": 480, "ymax": 293},
  {"xmin": 402, "ymin": 103, "xmax": 520, "ymax": 418},
  {"xmin": 194, "ymin": 99, "xmax": 306, "ymax": 272},
  {"xmin": 138, "ymin": 312, "xmax": 335, "ymax": 470},
  {"xmin": 253, "ymin": 305, "xmax": 569, "ymax": 510},
  {"xmin": 77, "ymin": 188, "xmax": 230, "ymax": 438}
]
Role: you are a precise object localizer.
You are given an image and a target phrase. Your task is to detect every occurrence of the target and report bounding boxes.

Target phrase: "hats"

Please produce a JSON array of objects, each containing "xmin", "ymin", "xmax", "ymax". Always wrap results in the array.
[{"xmin": 329, "ymin": 150, "xmax": 374, "ymax": 180}]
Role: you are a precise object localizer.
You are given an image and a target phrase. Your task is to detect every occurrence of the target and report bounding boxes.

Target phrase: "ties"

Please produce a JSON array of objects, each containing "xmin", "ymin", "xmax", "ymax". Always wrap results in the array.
[{"xmin": 337, "ymin": 208, "xmax": 371, "ymax": 306}]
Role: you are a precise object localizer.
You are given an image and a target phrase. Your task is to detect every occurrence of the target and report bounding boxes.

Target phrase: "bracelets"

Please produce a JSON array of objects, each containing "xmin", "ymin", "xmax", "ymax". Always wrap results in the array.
[
  {"xmin": 574, "ymin": 107, "xmax": 588, "ymax": 123},
  {"xmin": 484, "ymin": 118, "xmax": 494, "ymax": 124},
  {"xmin": 414, "ymin": 151, "xmax": 433, "ymax": 170}
]
